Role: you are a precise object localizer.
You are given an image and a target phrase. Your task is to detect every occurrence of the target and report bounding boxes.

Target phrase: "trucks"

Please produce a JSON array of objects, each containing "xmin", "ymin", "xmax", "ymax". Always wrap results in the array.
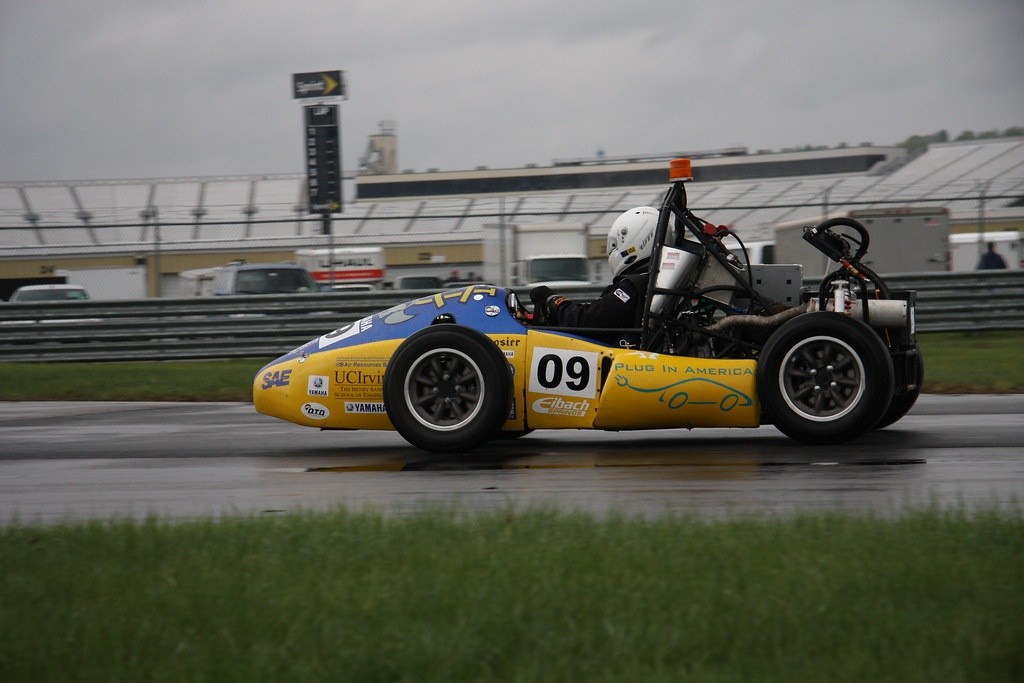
[
  {"xmin": 722, "ymin": 206, "xmax": 952, "ymax": 272},
  {"xmin": 479, "ymin": 220, "xmax": 596, "ymax": 296}
]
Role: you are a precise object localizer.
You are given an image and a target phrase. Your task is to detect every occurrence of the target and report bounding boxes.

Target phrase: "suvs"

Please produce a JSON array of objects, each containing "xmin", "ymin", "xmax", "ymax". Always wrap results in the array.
[{"xmin": 207, "ymin": 260, "xmax": 335, "ymax": 316}]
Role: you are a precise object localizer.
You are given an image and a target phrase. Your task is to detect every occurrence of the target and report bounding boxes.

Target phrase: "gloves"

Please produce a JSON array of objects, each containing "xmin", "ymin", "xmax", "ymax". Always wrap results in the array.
[{"xmin": 530, "ymin": 285, "xmax": 574, "ymax": 325}]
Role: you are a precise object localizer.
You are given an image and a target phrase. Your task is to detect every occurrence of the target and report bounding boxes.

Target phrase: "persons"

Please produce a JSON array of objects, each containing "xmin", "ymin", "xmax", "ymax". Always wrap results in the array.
[
  {"xmin": 529, "ymin": 205, "xmax": 674, "ymax": 329},
  {"xmin": 446, "ymin": 268, "xmax": 477, "ymax": 287},
  {"xmin": 978, "ymin": 241, "xmax": 1006, "ymax": 269}
]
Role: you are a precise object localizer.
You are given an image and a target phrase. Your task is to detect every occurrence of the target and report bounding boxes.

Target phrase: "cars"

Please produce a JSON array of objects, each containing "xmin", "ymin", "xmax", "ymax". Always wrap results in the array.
[
  {"xmin": 1, "ymin": 284, "xmax": 103, "ymax": 323},
  {"xmin": 252, "ymin": 159, "xmax": 925, "ymax": 462}
]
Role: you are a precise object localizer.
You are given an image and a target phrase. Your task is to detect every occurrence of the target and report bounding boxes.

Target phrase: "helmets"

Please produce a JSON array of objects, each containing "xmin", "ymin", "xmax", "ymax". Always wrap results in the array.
[{"xmin": 607, "ymin": 206, "xmax": 675, "ymax": 277}]
